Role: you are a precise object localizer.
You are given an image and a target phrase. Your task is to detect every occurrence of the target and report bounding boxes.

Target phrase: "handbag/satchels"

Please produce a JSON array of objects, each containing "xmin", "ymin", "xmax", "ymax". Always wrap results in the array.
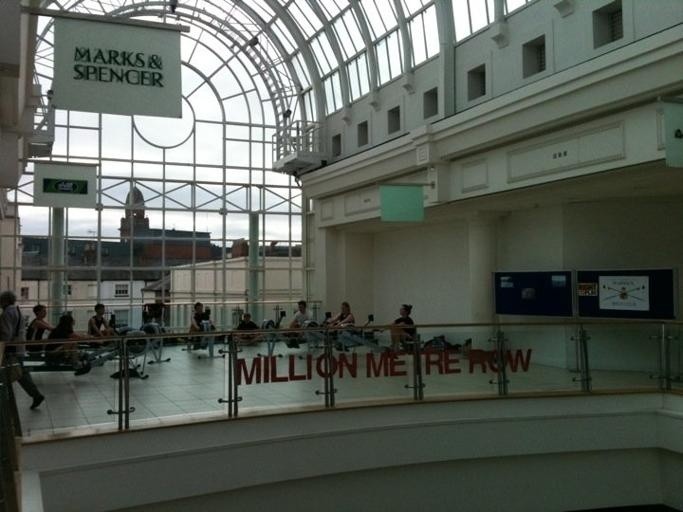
[{"xmin": 0, "ymin": 353, "xmax": 23, "ymax": 383}]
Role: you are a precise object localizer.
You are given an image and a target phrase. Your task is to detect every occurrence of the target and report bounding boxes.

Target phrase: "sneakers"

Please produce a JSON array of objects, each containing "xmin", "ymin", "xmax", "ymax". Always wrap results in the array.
[{"xmin": 30, "ymin": 393, "xmax": 44, "ymax": 410}]
